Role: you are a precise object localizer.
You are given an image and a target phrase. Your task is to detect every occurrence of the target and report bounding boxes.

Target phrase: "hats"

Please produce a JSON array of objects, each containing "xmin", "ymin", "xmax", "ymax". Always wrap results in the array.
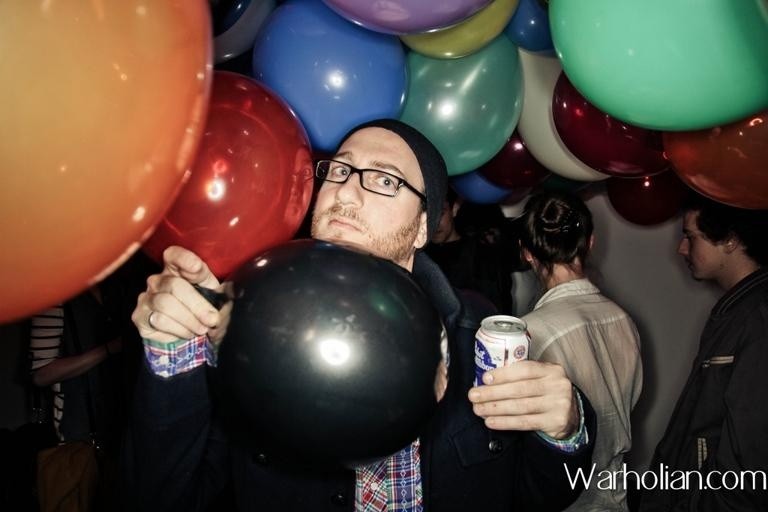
[{"xmin": 332, "ymin": 117, "xmax": 451, "ymax": 245}]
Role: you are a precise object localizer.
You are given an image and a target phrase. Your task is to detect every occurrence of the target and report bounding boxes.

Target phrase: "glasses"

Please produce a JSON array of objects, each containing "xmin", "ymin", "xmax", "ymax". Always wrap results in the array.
[{"xmin": 312, "ymin": 158, "xmax": 429, "ymax": 205}]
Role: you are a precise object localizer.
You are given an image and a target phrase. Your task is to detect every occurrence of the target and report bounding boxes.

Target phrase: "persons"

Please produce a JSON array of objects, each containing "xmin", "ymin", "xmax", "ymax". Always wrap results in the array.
[
  {"xmin": 131, "ymin": 119, "xmax": 597, "ymax": 511},
  {"xmin": 517, "ymin": 180, "xmax": 647, "ymax": 511},
  {"xmin": 639, "ymin": 206, "xmax": 766, "ymax": 510},
  {"xmin": 427, "ymin": 188, "xmax": 467, "ymax": 289},
  {"xmin": 27, "ymin": 288, "xmax": 132, "ymax": 510}
]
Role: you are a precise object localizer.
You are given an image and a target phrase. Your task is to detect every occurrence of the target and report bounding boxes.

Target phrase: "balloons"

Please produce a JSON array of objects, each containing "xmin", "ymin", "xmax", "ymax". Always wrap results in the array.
[
  {"xmin": 0, "ymin": 0, "xmax": 767, "ymax": 323},
  {"xmin": 228, "ymin": 241, "xmax": 452, "ymax": 463}
]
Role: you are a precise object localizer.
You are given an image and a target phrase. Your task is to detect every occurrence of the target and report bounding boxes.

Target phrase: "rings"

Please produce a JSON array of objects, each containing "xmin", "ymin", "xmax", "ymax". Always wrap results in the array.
[{"xmin": 147, "ymin": 312, "xmax": 156, "ymax": 328}]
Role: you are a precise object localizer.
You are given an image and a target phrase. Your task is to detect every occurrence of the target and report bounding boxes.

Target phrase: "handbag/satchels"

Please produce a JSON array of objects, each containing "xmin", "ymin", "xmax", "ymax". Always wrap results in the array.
[{"xmin": 34, "ymin": 436, "xmax": 101, "ymax": 512}]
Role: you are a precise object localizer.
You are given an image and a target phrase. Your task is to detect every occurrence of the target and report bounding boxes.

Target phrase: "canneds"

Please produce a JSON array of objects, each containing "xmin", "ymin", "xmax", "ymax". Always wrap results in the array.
[{"xmin": 473, "ymin": 315, "xmax": 531, "ymax": 387}]
